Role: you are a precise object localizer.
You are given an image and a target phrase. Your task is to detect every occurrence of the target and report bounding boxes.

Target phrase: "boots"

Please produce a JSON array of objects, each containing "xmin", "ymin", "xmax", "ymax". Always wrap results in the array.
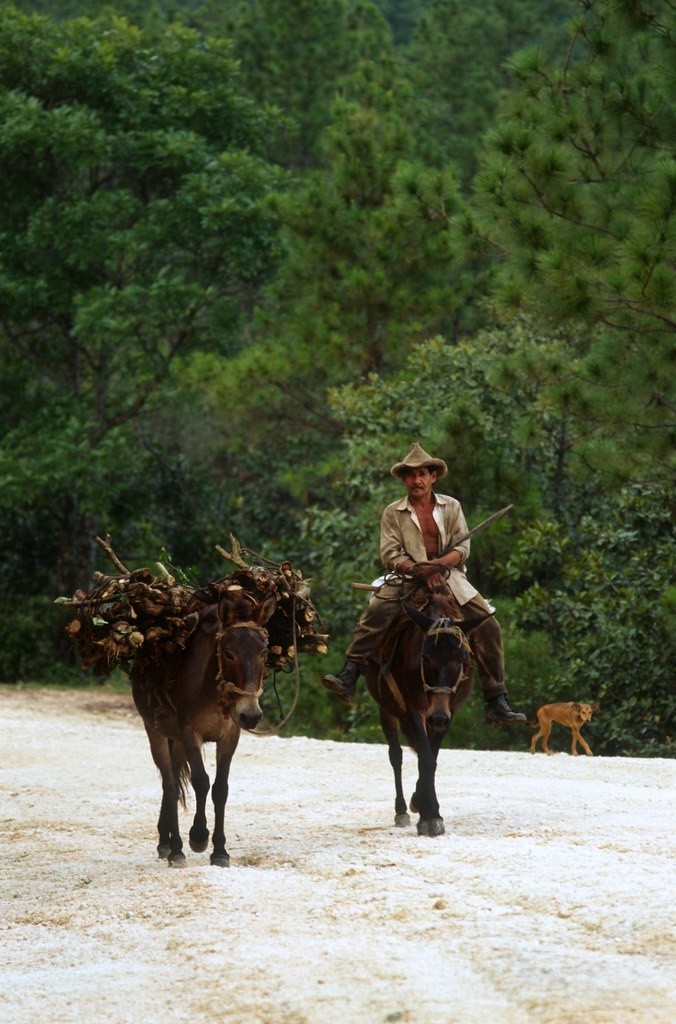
[
  {"xmin": 484, "ymin": 695, "xmax": 526, "ymax": 725},
  {"xmin": 323, "ymin": 658, "xmax": 364, "ymax": 703}
]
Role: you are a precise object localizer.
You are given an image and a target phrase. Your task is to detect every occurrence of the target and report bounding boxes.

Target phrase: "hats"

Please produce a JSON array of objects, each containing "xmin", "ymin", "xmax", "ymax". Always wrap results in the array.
[{"xmin": 391, "ymin": 442, "xmax": 447, "ymax": 477}]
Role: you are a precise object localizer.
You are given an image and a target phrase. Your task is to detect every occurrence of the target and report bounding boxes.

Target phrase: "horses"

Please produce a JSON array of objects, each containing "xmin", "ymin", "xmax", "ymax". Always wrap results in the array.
[
  {"xmin": 362, "ymin": 596, "xmax": 496, "ymax": 838},
  {"xmin": 126, "ymin": 581, "xmax": 273, "ymax": 868}
]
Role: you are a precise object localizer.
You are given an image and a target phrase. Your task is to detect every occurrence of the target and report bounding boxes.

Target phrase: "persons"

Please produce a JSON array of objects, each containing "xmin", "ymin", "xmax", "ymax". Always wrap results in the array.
[{"xmin": 321, "ymin": 442, "xmax": 526, "ymax": 725}]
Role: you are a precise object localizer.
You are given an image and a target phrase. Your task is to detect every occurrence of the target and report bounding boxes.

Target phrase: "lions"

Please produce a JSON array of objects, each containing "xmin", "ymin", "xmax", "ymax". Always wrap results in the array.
[{"xmin": 529, "ymin": 700, "xmax": 601, "ymax": 757}]
